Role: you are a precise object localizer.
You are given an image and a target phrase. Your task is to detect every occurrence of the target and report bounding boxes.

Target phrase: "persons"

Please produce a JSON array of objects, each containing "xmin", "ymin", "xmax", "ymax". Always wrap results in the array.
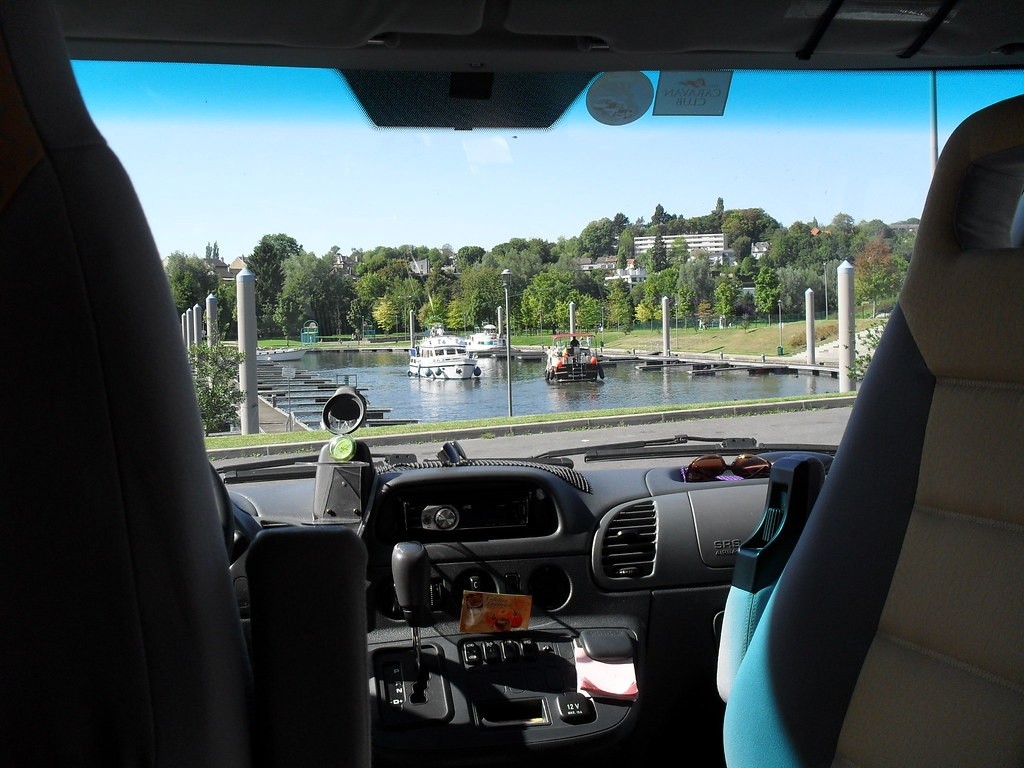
[{"xmin": 571, "ymin": 336, "xmax": 580, "ymax": 347}]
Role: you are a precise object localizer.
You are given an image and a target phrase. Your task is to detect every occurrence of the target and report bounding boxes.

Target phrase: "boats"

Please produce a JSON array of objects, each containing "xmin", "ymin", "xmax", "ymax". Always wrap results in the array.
[
  {"xmin": 544, "ymin": 332, "xmax": 600, "ymax": 384},
  {"xmin": 408, "ymin": 334, "xmax": 478, "ymax": 380},
  {"xmin": 465, "ymin": 331, "xmax": 521, "ymax": 359}
]
